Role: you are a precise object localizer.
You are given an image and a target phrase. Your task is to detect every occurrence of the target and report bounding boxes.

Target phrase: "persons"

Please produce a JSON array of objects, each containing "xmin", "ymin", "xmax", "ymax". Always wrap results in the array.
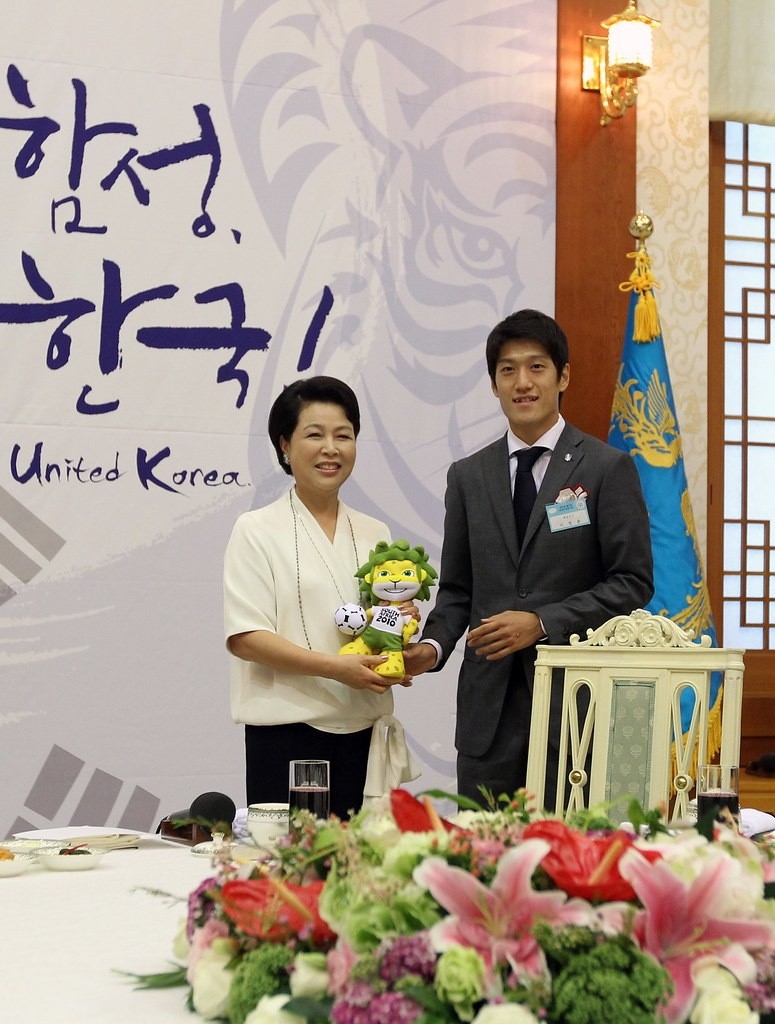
[
  {"xmin": 391, "ymin": 307, "xmax": 657, "ymax": 811},
  {"xmin": 222, "ymin": 376, "xmax": 423, "ymax": 817}
]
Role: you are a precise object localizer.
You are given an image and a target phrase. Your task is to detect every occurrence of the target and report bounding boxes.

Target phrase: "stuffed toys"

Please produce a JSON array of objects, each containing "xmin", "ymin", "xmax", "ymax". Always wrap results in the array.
[{"xmin": 337, "ymin": 539, "xmax": 438, "ymax": 678}]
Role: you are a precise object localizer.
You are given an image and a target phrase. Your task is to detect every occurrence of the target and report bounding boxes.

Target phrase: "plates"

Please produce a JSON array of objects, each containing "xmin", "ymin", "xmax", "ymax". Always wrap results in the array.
[{"xmin": 0, "ymin": 837, "xmax": 74, "ymax": 857}]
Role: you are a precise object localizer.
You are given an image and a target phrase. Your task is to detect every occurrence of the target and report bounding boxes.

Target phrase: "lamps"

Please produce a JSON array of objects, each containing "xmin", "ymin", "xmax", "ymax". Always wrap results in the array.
[{"xmin": 578, "ymin": 1, "xmax": 658, "ymax": 131}]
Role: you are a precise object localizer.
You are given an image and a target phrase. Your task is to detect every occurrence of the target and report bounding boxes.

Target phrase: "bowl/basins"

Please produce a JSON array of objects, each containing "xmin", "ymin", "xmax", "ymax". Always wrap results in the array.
[
  {"xmin": 0, "ymin": 852, "xmax": 39, "ymax": 878},
  {"xmin": 28, "ymin": 845, "xmax": 111, "ymax": 872}
]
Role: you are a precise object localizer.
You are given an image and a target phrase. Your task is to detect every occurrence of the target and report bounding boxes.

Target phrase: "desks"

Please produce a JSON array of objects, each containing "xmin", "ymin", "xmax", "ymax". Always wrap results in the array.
[{"xmin": 0, "ymin": 814, "xmax": 775, "ymax": 1024}]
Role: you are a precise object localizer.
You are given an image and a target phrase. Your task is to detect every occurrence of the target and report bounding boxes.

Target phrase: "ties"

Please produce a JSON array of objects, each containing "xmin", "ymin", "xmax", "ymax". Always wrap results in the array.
[{"xmin": 511, "ymin": 446, "xmax": 548, "ymax": 558}]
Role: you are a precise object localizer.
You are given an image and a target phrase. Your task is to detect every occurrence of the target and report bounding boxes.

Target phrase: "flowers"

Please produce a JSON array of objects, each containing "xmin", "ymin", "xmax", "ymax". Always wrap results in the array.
[{"xmin": 182, "ymin": 788, "xmax": 773, "ymax": 1024}]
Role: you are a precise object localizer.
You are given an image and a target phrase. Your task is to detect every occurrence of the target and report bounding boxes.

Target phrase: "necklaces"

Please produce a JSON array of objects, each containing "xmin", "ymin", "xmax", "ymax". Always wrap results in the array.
[{"xmin": 286, "ymin": 491, "xmax": 374, "ymax": 655}]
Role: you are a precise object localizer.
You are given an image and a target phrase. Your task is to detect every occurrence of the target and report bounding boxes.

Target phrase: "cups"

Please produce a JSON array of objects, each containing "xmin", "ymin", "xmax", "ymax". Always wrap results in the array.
[
  {"xmin": 286, "ymin": 759, "xmax": 331, "ymax": 839},
  {"xmin": 246, "ymin": 803, "xmax": 292, "ymax": 852},
  {"xmin": 696, "ymin": 763, "xmax": 742, "ymax": 844}
]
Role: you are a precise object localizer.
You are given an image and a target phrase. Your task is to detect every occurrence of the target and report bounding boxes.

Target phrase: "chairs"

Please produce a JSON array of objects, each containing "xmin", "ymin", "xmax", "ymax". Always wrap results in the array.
[{"xmin": 518, "ymin": 608, "xmax": 748, "ymax": 803}]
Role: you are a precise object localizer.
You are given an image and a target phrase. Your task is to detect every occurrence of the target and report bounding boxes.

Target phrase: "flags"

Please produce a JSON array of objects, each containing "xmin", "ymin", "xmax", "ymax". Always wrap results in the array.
[{"xmin": 598, "ymin": 247, "xmax": 731, "ymax": 799}]
